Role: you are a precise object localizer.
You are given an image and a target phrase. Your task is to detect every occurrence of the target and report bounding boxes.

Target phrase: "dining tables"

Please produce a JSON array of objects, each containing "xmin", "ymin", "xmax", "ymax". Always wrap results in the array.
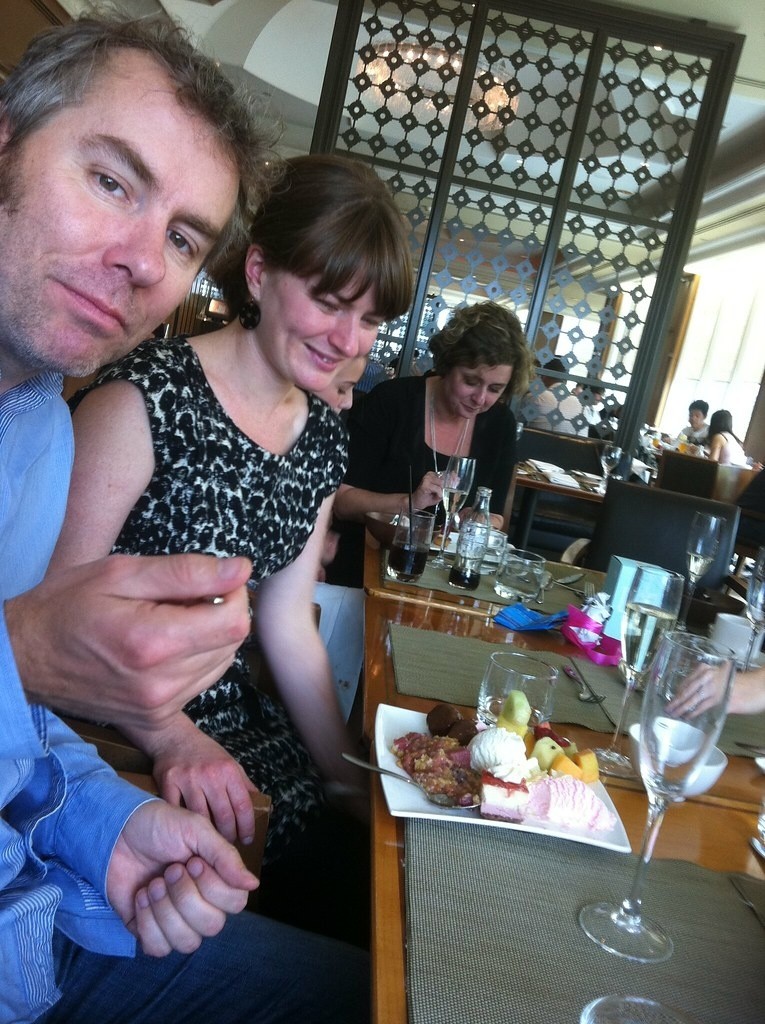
[
  {"xmin": 347, "ymin": 512, "xmax": 765, "ymax": 1024},
  {"xmin": 639, "ymin": 423, "xmax": 764, "ymax": 470}
]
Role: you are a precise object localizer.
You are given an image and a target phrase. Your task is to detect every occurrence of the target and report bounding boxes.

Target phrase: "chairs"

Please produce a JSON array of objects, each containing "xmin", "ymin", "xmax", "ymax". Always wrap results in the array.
[
  {"xmin": 559, "ymin": 477, "xmax": 742, "ymax": 613},
  {"xmin": 656, "ymin": 449, "xmax": 719, "ymax": 500},
  {"xmin": 511, "ymin": 426, "xmax": 604, "ymax": 538},
  {"xmin": 716, "ymin": 463, "xmax": 762, "ymax": 595}
]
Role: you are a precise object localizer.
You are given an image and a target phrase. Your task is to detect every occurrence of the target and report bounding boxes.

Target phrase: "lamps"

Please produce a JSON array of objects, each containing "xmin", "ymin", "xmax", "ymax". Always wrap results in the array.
[{"xmin": 354, "ymin": 32, "xmax": 523, "ymax": 133}]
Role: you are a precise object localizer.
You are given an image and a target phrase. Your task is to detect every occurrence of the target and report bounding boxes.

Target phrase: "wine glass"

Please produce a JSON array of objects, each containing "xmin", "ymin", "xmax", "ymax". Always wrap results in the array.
[
  {"xmin": 672, "ymin": 513, "xmax": 727, "ymax": 631},
  {"xmin": 741, "ymin": 546, "xmax": 765, "ymax": 673},
  {"xmin": 425, "ymin": 455, "xmax": 477, "ymax": 570},
  {"xmin": 579, "ymin": 631, "xmax": 736, "ymax": 963},
  {"xmin": 598, "ymin": 444, "xmax": 622, "ymax": 488},
  {"xmin": 592, "ymin": 565, "xmax": 685, "ymax": 779}
]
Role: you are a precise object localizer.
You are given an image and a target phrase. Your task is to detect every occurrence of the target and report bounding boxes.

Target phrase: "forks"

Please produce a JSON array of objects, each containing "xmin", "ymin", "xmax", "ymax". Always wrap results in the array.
[
  {"xmin": 584, "ymin": 581, "xmax": 595, "ymax": 601},
  {"xmin": 564, "ymin": 665, "xmax": 608, "ymax": 704}
]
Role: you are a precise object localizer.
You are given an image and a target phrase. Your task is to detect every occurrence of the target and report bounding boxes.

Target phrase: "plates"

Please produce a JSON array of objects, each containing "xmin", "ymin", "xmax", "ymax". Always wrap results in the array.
[
  {"xmin": 429, "ymin": 529, "xmax": 515, "ymax": 564},
  {"xmin": 755, "ymin": 757, "xmax": 765, "ymax": 775},
  {"xmin": 525, "ymin": 459, "xmax": 566, "ymax": 473},
  {"xmin": 572, "ymin": 470, "xmax": 603, "ymax": 481},
  {"xmin": 736, "ymin": 648, "xmax": 764, "ymax": 669},
  {"xmin": 375, "ymin": 702, "xmax": 631, "ymax": 853}
]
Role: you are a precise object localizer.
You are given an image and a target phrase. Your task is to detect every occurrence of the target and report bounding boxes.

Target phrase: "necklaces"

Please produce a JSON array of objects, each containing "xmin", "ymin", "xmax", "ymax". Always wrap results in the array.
[{"xmin": 430, "ymin": 378, "xmax": 470, "ymax": 517}]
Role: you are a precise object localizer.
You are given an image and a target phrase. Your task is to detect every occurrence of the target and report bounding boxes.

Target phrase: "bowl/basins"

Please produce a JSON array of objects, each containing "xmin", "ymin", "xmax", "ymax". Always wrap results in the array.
[
  {"xmin": 627, "ymin": 722, "xmax": 727, "ymax": 802},
  {"xmin": 652, "ymin": 715, "xmax": 705, "ymax": 769},
  {"xmin": 365, "ymin": 511, "xmax": 442, "ymax": 550}
]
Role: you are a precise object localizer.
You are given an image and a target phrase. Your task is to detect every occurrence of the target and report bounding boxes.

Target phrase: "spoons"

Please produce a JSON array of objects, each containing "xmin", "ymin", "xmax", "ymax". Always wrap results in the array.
[
  {"xmin": 342, "ymin": 753, "xmax": 480, "ymax": 808},
  {"xmin": 552, "ymin": 573, "xmax": 586, "ymax": 586}
]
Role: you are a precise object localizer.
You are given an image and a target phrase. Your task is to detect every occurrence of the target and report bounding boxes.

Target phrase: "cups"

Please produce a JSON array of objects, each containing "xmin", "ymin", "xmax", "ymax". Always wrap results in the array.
[
  {"xmin": 387, "ymin": 507, "xmax": 435, "ymax": 584},
  {"xmin": 494, "ymin": 547, "xmax": 547, "ymax": 604},
  {"xmin": 653, "ymin": 433, "xmax": 662, "ymax": 447},
  {"xmin": 757, "ymin": 799, "xmax": 765, "ymax": 843},
  {"xmin": 678, "ymin": 439, "xmax": 689, "ymax": 454},
  {"xmin": 474, "ymin": 649, "xmax": 559, "ymax": 735},
  {"xmin": 710, "ymin": 612, "xmax": 753, "ymax": 661},
  {"xmin": 476, "ymin": 529, "xmax": 506, "ymax": 576}
]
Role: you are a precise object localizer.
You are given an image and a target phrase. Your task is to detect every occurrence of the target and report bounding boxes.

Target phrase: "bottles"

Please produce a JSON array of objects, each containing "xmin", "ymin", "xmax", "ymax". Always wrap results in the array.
[{"xmin": 447, "ymin": 487, "xmax": 493, "ymax": 590}]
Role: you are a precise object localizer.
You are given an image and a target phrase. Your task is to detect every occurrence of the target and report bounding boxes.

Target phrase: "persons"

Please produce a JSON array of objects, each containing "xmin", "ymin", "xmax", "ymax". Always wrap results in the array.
[
  {"xmin": 391, "ymin": 348, "xmax": 419, "ymax": 372},
  {"xmin": 44, "ymin": 153, "xmax": 417, "ymax": 943},
  {"xmin": 707, "ymin": 410, "xmax": 746, "ymax": 465},
  {"xmin": 311, "ymin": 354, "xmax": 367, "ymax": 582},
  {"xmin": 335, "ymin": 301, "xmax": 528, "ymax": 589},
  {"xmin": 527, "ymin": 358, "xmax": 606, "ymax": 438},
  {"xmin": 682, "ymin": 399, "xmax": 710, "ymax": 445},
  {"xmin": 0, "ymin": 0, "xmax": 376, "ymax": 1024}
]
